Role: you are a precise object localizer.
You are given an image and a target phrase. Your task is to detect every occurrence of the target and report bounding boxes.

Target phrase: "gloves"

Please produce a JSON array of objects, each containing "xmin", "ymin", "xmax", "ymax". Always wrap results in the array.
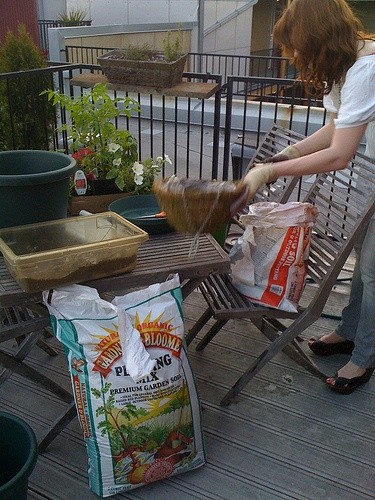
[
  {"xmin": 229, "ymin": 161, "xmax": 276, "ymax": 214},
  {"xmin": 248, "ymin": 145, "xmax": 301, "ymax": 186}
]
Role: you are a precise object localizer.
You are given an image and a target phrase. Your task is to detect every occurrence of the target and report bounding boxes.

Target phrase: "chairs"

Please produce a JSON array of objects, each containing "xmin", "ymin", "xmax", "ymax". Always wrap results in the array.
[
  {"xmin": 181, "ymin": 122, "xmax": 307, "ymax": 350},
  {"xmin": 0, "ymin": 299, "xmax": 73, "ymax": 404},
  {"xmin": 193, "ymin": 151, "xmax": 375, "ymax": 409}
]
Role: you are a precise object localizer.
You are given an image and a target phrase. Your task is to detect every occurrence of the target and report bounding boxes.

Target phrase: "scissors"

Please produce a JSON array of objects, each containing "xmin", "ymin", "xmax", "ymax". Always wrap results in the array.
[{"xmin": 137, "ymin": 212, "xmax": 165, "ymax": 219}]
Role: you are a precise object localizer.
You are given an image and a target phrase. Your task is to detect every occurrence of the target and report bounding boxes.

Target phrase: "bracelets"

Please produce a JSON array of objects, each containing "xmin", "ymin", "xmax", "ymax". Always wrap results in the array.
[
  {"xmin": 282, "ymin": 146, "xmax": 301, "ymax": 160},
  {"xmin": 262, "ymin": 162, "xmax": 274, "ymax": 180}
]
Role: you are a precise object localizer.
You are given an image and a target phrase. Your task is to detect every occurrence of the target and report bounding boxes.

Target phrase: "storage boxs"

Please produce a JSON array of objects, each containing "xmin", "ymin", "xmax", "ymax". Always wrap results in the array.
[
  {"xmin": 68, "ymin": 193, "xmax": 130, "ymax": 216},
  {"xmin": 0, "ymin": 209, "xmax": 149, "ymax": 296}
]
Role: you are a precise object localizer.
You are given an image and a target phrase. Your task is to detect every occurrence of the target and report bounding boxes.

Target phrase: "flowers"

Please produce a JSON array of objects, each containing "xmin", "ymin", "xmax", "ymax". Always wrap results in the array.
[{"xmin": 39, "ymin": 81, "xmax": 174, "ymax": 200}]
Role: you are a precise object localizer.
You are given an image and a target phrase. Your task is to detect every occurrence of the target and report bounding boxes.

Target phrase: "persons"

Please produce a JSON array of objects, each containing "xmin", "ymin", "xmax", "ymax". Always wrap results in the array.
[{"xmin": 229, "ymin": 0, "xmax": 375, "ymax": 395}]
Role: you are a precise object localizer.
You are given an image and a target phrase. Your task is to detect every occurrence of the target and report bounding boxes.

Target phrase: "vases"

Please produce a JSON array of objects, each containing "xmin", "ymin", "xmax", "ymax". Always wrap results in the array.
[
  {"xmin": 0, "ymin": 150, "xmax": 79, "ymax": 260},
  {"xmin": 71, "ymin": 176, "xmax": 129, "ymax": 196}
]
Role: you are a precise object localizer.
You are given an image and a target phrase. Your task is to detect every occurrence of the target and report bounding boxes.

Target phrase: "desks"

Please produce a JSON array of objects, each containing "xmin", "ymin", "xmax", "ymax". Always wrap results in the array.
[
  {"xmin": 70, "ymin": 74, "xmax": 222, "ymax": 100},
  {"xmin": 0, "ymin": 226, "xmax": 231, "ymax": 458}
]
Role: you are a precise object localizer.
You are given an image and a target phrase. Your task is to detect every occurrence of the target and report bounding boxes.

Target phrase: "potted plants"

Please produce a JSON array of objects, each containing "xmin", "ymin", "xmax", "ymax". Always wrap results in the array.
[
  {"xmin": 54, "ymin": 5, "xmax": 89, "ymax": 27},
  {"xmin": 97, "ymin": 16, "xmax": 189, "ymax": 88}
]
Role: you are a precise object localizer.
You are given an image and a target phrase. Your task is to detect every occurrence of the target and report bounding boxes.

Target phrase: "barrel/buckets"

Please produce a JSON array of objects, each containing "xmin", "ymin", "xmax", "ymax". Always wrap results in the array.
[
  {"xmin": 108, "ymin": 194, "xmax": 175, "ymax": 237},
  {"xmin": 0, "ymin": 150, "xmax": 78, "ymax": 259},
  {"xmin": 0, "ymin": 410, "xmax": 38, "ymax": 500}
]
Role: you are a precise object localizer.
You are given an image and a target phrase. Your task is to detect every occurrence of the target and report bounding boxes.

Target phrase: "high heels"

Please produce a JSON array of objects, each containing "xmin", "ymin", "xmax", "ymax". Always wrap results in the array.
[
  {"xmin": 327, "ymin": 361, "xmax": 375, "ymax": 395},
  {"xmin": 307, "ymin": 337, "xmax": 355, "ymax": 356}
]
